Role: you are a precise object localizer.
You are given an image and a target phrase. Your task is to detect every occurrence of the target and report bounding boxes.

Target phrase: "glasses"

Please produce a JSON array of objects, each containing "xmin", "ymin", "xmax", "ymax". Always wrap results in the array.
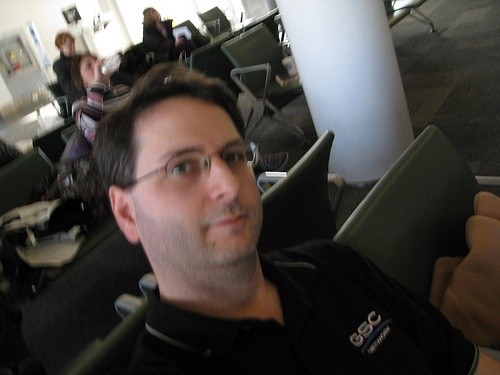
[{"xmin": 120, "ymin": 141, "xmax": 252, "ymax": 190}]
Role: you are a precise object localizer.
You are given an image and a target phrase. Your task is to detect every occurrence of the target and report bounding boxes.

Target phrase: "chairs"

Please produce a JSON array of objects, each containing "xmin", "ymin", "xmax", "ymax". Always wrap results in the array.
[
  {"xmin": 139, "ymin": 131, "xmax": 334, "ymax": 295},
  {"xmin": 333, "ymin": 125, "xmax": 478, "ymax": 299},
  {"xmin": 383, "ymin": 0, "xmax": 436, "ymax": 36},
  {"xmin": 67, "ymin": 293, "xmax": 165, "ymax": 375},
  {"xmin": 174, "ymin": 6, "xmax": 306, "ymax": 145}
]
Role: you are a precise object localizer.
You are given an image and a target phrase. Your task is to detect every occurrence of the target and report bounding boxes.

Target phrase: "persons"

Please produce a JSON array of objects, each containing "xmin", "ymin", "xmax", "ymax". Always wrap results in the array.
[
  {"xmin": 143, "ymin": 8, "xmax": 183, "ymax": 62},
  {"xmin": 53, "ymin": 32, "xmax": 87, "ymax": 102},
  {"xmin": 71, "ymin": 54, "xmax": 130, "ymax": 145},
  {"xmin": 93, "ymin": 61, "xmax": 500, "ymax": 374}
]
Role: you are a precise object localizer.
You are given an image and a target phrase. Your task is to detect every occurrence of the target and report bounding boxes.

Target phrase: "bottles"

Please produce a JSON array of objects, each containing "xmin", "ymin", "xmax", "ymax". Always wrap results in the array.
[{"xmin": 99, "ymin": 54, "xmax": 121, "ymax": 76}]
